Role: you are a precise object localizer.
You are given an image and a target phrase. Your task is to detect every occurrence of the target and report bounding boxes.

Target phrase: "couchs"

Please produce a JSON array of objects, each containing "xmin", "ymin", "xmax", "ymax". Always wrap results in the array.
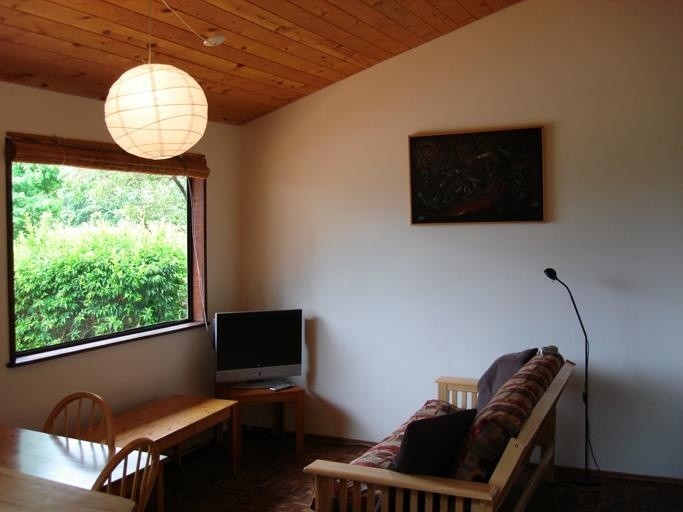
[{"xmin": 302, "ymin": 344, "xmax": 576, "ymax": 512}]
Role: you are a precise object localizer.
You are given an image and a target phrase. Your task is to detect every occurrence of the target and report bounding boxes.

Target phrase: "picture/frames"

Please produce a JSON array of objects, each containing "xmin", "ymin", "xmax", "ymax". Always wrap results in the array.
[{"xmin": 408, "ymin": 125, "xmax": 547, "ymax": 224}]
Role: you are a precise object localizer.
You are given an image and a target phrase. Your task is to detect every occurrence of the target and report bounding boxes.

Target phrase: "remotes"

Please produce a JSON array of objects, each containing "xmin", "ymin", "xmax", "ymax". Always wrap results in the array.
[{"xmin": 267, "ymin": 382, "xmax": 297, "ymax": 392}]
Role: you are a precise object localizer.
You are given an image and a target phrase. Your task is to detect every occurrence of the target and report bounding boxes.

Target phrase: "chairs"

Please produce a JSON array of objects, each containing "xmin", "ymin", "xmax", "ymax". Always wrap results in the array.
[
  {"xmin": 42, "ymin": 391, "xmax": 116, "ymax": 446},
  {"xmin": 91, "ymin": 437, "xmax": 160, "ymax": 512}
]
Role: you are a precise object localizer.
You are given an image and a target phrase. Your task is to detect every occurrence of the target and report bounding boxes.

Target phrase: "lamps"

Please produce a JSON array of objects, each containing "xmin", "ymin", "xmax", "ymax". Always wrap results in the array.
[{"xmin": 104, "ymin": 62, "xmax": 208, "ymax": 162}]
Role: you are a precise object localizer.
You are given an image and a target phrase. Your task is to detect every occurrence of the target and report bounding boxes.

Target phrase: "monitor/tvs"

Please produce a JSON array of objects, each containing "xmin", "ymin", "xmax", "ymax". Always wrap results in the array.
[{"xmin": 214, "ymin": 308, "xmax": 303, "ymax": 391}]
